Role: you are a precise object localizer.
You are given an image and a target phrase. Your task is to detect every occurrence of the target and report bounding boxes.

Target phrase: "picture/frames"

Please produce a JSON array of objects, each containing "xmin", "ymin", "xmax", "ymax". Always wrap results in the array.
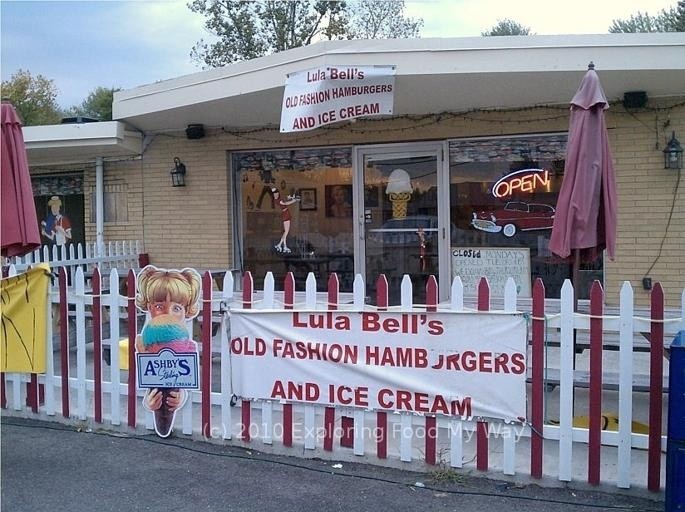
[
  {"xmin": 299, "ymin": 187, "xmax": 317, "ymax": 211},
  {"xmin": 325, "ymin": 185, "xmax": 353, "ymax": 218}
]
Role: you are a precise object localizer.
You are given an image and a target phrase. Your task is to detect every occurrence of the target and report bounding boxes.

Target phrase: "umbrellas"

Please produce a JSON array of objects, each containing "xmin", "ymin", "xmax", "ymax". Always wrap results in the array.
[
  {"xmin": 0, "ymin": 95, "xmax": 42, "ymax": 260},
  {"xmin": 547, "ymin": 59, "xmax": 620, "ymax": 314}
]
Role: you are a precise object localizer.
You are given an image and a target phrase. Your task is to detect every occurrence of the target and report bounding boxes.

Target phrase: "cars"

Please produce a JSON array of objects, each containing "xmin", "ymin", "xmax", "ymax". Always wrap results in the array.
[
  {"xmin": 471, "ymin": 201, "xmax": 555, "ymax": 238},
  {"xmin": 376, "ymin": 216, "xmax": 438, "ymax": 243}
]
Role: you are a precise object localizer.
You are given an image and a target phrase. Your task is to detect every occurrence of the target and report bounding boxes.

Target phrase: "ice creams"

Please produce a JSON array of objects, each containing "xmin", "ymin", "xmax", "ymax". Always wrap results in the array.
[
  {"xmin": 141, "ymin": 314, "xmax": 200, "ymax": 437},
  {"xmin": 386, "ymin": 168, "xmax": 413, "ymax": 219}
]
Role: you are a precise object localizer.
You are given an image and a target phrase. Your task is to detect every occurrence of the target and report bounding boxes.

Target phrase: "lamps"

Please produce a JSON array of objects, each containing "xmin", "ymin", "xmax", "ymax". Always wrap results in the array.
[
  {"xmin": 170, "ymin": 156, "xmax": 187, "ymax": 189},
  {"xmin": 662, "ymin": 134, "xmax": 685, "ymax": 171}
]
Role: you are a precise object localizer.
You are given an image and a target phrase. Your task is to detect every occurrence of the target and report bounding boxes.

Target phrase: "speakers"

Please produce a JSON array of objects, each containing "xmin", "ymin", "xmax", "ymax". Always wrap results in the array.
[
  {"xmin": 624, "ymin": 91, "xmax": 648, "ymax": 109},
  {"xmin": 186, "ymin": 124, "xmax": 204, "ymax": 140}
]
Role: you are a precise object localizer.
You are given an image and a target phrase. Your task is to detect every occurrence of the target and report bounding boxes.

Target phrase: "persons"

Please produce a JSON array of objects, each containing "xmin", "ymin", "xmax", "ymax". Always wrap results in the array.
[
  {"xmin": 329, "ymin": 185, "xmax": 351, "ymax": 217},
  {"xmin": 39, "ymin": 195, "xmax": 74, "ymax": 247},
  {"xmin": 302, "ymin": 191, "xmax": 312, "ymax": 205},
  {"xmin": 271, "ymin": 186, "xmax": 298, "ymax": 254},
  {"xmin": 129, "ymin": 265, "xmax": 203, "ymax": 416},
  {"xmin": 364, "ymin": 188, "xmax": 376, "ymax": 207}
]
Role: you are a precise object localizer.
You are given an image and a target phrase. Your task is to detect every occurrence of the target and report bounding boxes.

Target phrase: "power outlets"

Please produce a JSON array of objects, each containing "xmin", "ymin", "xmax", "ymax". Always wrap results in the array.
[{"xmin": 643, "ymin": 278, "xmax": 652, "ymax": 289}]
